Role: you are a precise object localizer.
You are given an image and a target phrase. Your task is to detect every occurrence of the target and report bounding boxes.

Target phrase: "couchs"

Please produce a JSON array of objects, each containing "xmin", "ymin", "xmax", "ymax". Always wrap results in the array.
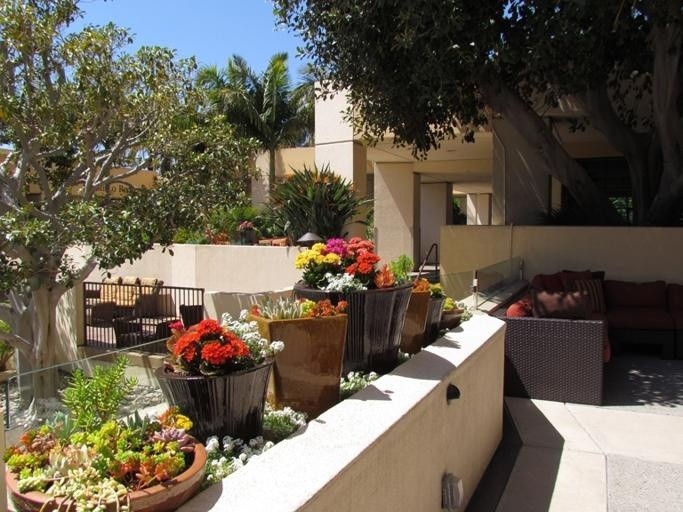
[
  {"xmin": 491, "ymin": 271, "xmax": 683, "ymax": 405},
  {"xmin": 85, "ymin": 276, "xmax": 163, "ymax": 317},
  {"xmin": 112, "ymin": 318, "xmax": 180, "ymax": 347}
]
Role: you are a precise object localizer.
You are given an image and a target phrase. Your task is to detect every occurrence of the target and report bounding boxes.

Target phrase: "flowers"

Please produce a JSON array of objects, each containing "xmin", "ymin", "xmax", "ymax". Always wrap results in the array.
[
  {"xmin": 165, "ymin": 310, "xmax": 284, "ymax": 376},
  {"xmin": 294, "ymin": 236, "xmax": 394, "ymax": 291}
]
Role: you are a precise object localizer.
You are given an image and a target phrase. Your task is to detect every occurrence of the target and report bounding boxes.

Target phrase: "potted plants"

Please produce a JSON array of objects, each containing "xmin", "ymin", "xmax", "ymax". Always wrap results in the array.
[
  {"xmin": 390, "ymin": 253, "xmax": 431, "ymax": 356},
  {"xmin": 443, "ymin": 298, "xmax": 462, "ymax": 330},
  {"xmin": 255, "ymin": 293, "xmax": 353, "ymax": 416},
  {"xmin": 427, "ymin": 282, "xmax": 444, "ymax": 344},
  {"xmin": 4, "ymin": 354, "xmax": 207, "ymax": 512}
]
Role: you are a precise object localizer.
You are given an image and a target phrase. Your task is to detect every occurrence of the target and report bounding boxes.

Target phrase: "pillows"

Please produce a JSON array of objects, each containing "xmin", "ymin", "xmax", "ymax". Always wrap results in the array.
[
  {"xmin": 571, "ymin": 278, "xmax": 606, "ymax": 313},
  {"xmin": 559, "ymin": 271, "xmax": 605, "ymax": 293},
  {"xmin": 529, "ymin": 289, "xmax": 587, "ymax": 318}
]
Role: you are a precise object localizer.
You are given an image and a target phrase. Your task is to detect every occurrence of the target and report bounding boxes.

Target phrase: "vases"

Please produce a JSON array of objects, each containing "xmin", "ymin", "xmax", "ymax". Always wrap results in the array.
[
  {"xmin": 291, "ymin": 280, "xmax": 412, "ymax": 381},
  {"xmin": 154, "ymin": 359, "xmax": 274, "ymax": 445}
]
